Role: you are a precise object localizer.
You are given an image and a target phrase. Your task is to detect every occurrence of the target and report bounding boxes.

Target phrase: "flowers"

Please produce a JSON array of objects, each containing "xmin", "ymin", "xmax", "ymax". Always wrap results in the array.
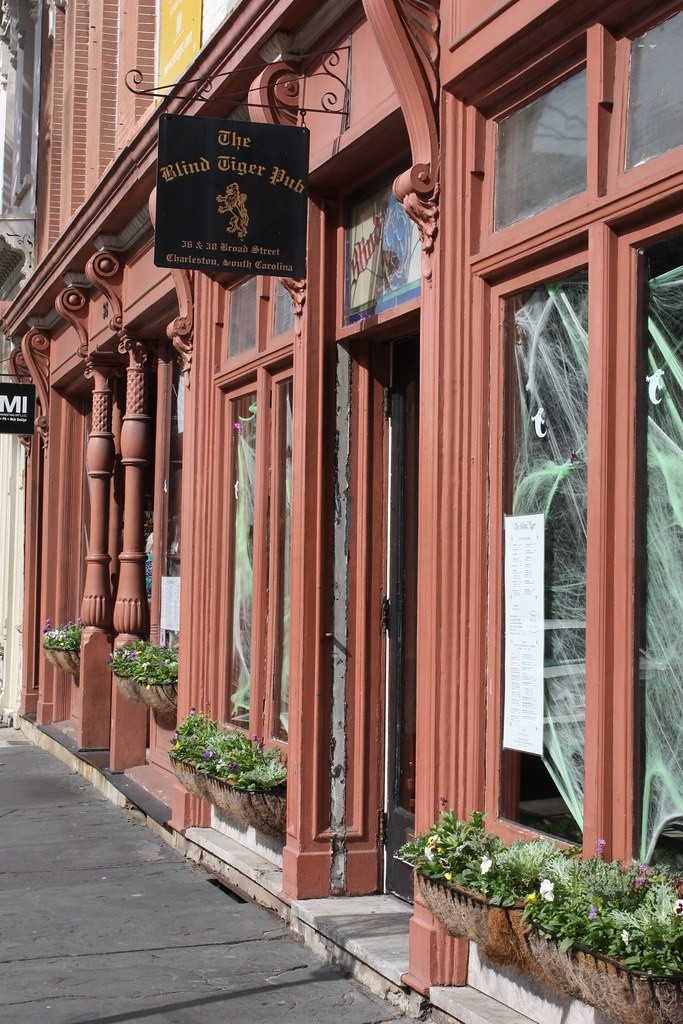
[
  {"xmin": 392, "ymin": 796, "xmax": 556, "ymax": 905},
  {"xmin": 105, "ymin": 640, "xmax": 180, "ymax": 684},
  {"xmin": 527, "ymin": 839, "xmax": 683, "ymax": 976},
  {"xmin": 42, "ymin": 616, "xmax": 84, "ymax": 649},
  {"xmin": 168, "ymin": 704, "xmax": 286, "ymax": 790}
]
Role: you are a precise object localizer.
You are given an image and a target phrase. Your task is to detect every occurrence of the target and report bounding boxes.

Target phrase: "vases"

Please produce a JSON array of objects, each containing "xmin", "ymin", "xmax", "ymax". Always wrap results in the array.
[
  {"xmin": 167, "ymin": 756, "xmax": 284, "ymax": 840},
  {"xmin": 42, "ymin": 646, "xmax": 80, "ymax": 677},
  {"xmin": 524, "ymin": 918, "xmax": 683, "ymax": 1023},
  {"xmin": 113, "ymin": 669, "xmax": 177, "ymax": 717},
  {"xmin": 414, "ymin": 870, "xmax": 525, "ymax": 966}
]
